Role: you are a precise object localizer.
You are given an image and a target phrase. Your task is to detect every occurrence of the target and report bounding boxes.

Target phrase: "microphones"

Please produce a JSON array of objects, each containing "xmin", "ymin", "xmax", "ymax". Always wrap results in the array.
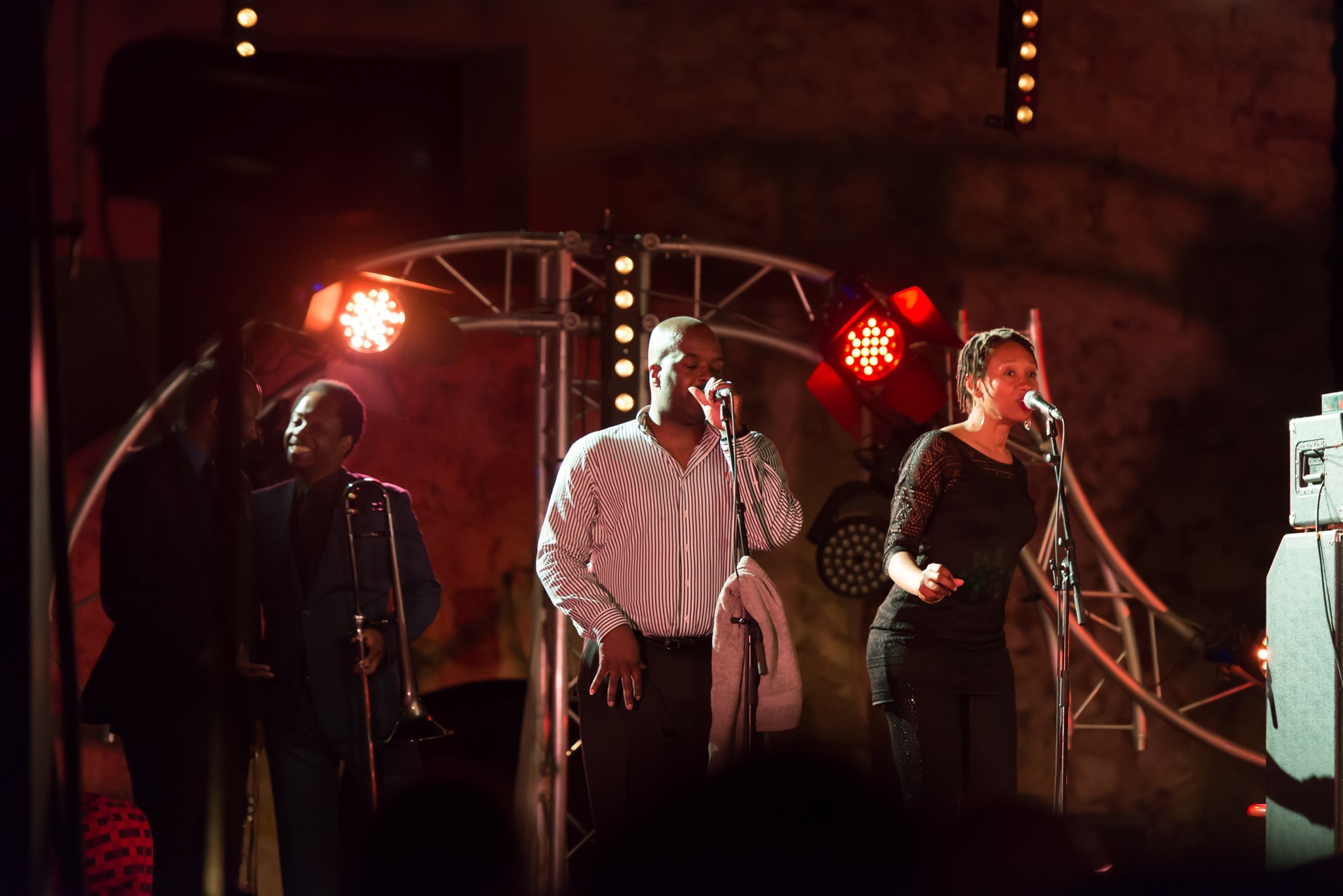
[
  {"xmin": 700, "ymin": 387, "xmax": 731, "ymax": 401},
  {"xmin": 1021, "ymin": 389, "xmax": 1064, "ymax": 421}
]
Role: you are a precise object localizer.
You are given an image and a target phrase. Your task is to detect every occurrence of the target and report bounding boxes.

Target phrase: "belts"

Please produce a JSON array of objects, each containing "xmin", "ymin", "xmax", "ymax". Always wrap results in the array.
[{"xmin": 632, "ymin": 633, "xmax": 713, "ymax": 652}]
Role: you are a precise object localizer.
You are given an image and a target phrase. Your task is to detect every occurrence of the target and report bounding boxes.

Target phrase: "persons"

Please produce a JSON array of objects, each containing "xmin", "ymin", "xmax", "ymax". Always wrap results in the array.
[
  {"xmin": 533, "ymin": 315, "xmax": 804, "ymax": 895},
  {"xmin": 232, "ymin": 377, "xmax": 442, "ymax": 896},
  {"xmin": 75, "ymin": 362, "xmax": 261, "ymax": 896},
  {"xmin": 865, "ymin": 327, "xmax": 1037, "ymax": 896}
]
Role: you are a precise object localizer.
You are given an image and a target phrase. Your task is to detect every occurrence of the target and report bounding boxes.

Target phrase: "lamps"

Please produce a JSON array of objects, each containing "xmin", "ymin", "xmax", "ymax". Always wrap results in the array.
[
  {"xmin": 807, "ymin": 286, "xmax": 948, "ymax": 447},
  {"xmin": 332, "ymin": 282, "xmax": 408, "ymax": 360}
]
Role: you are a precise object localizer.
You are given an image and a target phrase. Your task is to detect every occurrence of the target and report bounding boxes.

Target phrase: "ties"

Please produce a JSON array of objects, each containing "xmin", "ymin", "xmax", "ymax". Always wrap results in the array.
[{"xmin": 298, "ymin": 487, "xmax": 317, "ymax": 584}]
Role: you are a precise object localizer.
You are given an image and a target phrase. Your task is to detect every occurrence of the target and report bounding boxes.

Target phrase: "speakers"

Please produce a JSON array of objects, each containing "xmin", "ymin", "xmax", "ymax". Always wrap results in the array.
[
  {"xmin": 1262, "ymin": 531, "xmax": 1339, "ymax": 867},
  {"xmin": 1285, "ymin": 412, "xmax": 1343, "ymax": 526}
]
